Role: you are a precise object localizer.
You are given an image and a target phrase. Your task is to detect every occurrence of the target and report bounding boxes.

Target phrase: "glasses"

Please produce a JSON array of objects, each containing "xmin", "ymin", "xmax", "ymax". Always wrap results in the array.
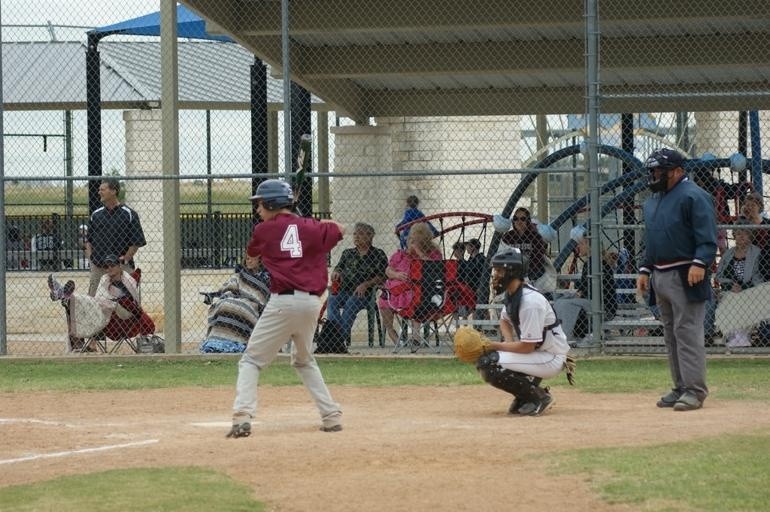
[{"xmin": 513, "ymin": 215, "xmax": 527, "ymax": 222}]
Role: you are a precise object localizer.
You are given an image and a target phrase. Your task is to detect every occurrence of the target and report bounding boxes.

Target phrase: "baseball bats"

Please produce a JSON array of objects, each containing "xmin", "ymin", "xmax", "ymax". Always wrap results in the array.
[{"xmin": 291, "ymin": 134, "xmax": 312, "ymax": 211}]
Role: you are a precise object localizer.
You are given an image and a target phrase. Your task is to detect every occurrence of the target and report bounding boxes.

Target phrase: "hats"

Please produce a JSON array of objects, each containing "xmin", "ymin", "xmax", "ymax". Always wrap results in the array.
[{"xmin": 104, "ymin": 254, "xmax": 117, "ymax": 262}]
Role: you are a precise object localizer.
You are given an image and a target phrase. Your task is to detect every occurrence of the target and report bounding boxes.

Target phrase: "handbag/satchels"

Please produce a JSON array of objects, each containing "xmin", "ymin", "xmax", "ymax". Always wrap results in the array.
[{"xmin": 314, "ymin": 319, "xmax": 347, "ymax": 354}]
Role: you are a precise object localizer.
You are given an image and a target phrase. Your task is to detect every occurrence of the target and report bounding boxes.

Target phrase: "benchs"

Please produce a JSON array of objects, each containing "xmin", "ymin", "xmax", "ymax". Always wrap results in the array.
[{"xmin": 462, "ymin": 271, "xmax": 723, "ymax": 346}]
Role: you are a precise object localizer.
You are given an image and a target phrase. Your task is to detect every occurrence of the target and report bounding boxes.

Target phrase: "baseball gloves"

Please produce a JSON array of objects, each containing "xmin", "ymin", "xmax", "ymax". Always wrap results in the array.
[{"xmin": 454, "ymin": 326, "xmax": 490, "ymax": 360}]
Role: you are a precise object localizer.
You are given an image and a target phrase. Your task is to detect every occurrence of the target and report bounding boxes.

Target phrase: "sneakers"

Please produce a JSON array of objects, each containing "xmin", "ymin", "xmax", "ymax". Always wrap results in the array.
[
  {"xmin": 508, "ymin": 386, "xmax": 555, "ymax": 415},
  {"xmin": 657, "ymin": 392, "xmax": 679, "ymax": 408},
  {"xmin": 61, "ymin": 280, "xmax": 74, "ymax": 307},
  {"xmin": 674, "ymin": 389, "xmax": 703, "ymax": 411},
  {"xmin": 48, "ymin": 275, "xmax": 62, "ymax": 301},
  {"xmin": 226, "ymin": 423, "xmax": 251, "ymax": 438}
]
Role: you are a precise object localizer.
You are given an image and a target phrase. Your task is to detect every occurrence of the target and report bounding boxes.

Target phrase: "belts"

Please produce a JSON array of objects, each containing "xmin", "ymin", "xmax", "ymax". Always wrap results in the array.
[{"xmin": 277, "ymin": 288, "xmax": 320, "ymax": 296}]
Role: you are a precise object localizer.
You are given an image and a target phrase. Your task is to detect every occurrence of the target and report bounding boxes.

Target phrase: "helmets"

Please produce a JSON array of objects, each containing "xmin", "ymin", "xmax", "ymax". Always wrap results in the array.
[
  {"xmin": 488, "ymin": 248, "xmax": 529, "ymax": 296},
  {"xmin": 247, "ymin": 178, "xmax": 295, "ymax": 211},
  {"xmin": 644, "ymin": 147, "xmax": 684, "ymax": 193}
]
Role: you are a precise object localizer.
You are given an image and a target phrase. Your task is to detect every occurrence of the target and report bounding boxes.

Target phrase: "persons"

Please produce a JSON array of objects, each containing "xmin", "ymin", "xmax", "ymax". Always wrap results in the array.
[
  {"xmin": 702, "ymin": 192, "xmax": 770, "ymax": 354},
  {"xmin": 327, "ymin": 222, "xmax": 389, "ymax": 353},
  {"xmin": 83, "ymin": 178, "xmax": 147, "ymax": 352},
  {"xmin": 9, "ymin": 218, "xmax": 91, "ymax": 272},
  {"xmin": 48, "ymin": 254, "xmax": 137, "ymax": 338},
  {"xmin": 636, "ymin": 149, "xmax": 719, "ymax": 412},
  {"xmin": 455, "ymin": 248, "xmax": 576, "ymax": 417},
  {"xmin": 200, "ymin": 254, "xmax": 271, "ymax": 353},
  {"xmin": 501, "ymin": 207, "xmax": 555, "ymax": 308},
  {"xmin": 573, "ymin": 231, "xmax": 618, "ymax": 346},
  {"xmin": 451, "ymin": 238, "xmax": 487, "ymax": 329},
  {"xmin": 225, "ymin": 178, "xmax": 348, "ymax": 439},
  {"xmin": 376, "ymin": 222, "xmax": 443, "ymax": 353},
  {"xmin": 394, "ymin": 195, "xmax": 439, "ymax": 249}
]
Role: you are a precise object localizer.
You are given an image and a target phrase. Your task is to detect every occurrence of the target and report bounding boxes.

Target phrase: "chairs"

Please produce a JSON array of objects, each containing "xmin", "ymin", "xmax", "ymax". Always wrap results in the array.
[
  {"xmin": 80, "ymin": 269, "xmax": 141, "ymax": 355},
  {"xmin": 381, "ymin": 261, "xmax": 457, "ymax": 355},
  {"xmin": 318, "ymin": 275, "xmax": 380, "ymax": 348}
]
